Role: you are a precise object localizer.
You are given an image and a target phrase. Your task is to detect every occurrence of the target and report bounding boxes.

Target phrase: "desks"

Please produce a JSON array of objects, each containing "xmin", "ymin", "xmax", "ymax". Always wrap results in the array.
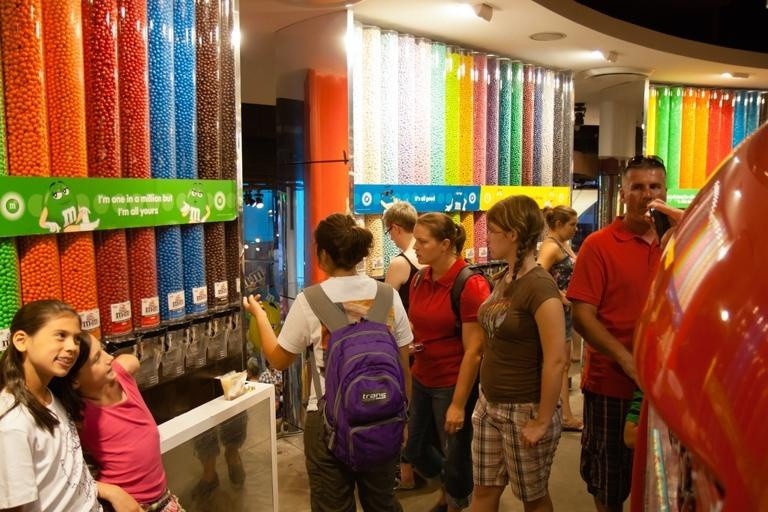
[{"xmin": 121, "ymin": 371, "xmax": 278, "ymax": 512}]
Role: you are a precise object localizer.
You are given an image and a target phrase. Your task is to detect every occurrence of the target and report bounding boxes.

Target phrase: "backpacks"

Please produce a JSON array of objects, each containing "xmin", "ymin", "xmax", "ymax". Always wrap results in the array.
[{"xmin": 301, "ymin": 281, "xmax": 411, "ymax": 476}]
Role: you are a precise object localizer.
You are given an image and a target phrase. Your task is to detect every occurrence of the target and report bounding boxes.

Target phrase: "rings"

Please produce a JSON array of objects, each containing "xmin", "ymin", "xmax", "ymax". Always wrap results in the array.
[{"xmin": 455, "ymin": 426, "xmax": 462, "ymax": 431}]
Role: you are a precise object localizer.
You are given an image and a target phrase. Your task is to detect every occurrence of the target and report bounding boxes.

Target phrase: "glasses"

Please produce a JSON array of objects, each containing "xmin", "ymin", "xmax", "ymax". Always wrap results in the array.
[
  {"xmin": 384, "ymin": 223, "xmax": 400, "ymax": 235},
  {"xmin": 627, "ymin": 155, "xmax": 664, "ymax": 169},
  {"xmin": 487, "ymin": 230, "xmax": 504, "ymax": 236}
]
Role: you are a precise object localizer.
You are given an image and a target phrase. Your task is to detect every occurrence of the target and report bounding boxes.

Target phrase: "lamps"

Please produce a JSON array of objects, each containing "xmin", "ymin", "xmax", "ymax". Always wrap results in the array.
[
  {"xmin": 608, "ymin": 52, "xmax": 617, "ymax": 62},
  {"xmin": 244, "ymin": 188, "xmax": 264, "ymax": 209},
  {"xmin": 471, "ymin": 2, "xmax": 495, "ymax": 22}
]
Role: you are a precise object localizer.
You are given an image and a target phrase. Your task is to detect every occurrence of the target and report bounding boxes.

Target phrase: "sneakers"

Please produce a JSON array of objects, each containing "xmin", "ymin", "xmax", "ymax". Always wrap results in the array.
[
  {"xmin": 561, "ymin": 420, "xmax": 584, "ymax": 431},
  {"xmin": 224, "ymin": 450, "xmax": 246, "ymax": 485},
  {"xmin": 394, "ymin": 471, "xmax": 441, "ymax": 498},
  {"xmin": 192, "ymin": 472, "xmax": 219, "ymax": 501}
]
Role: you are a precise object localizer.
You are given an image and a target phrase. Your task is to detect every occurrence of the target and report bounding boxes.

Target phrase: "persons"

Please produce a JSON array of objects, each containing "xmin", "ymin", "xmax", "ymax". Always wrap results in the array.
[
  {"xmin": 568, "ymin": 155, "xmax": 678, "ymax": 512},
  {"xmin": 467, "ymin": 195, "xmax": 568, "ymax": 511},
  {"xmin": 243, "ymin": 212, "xmax": 418, "ymax": 512},
  {"xmin": 408, "ymin": 212, "xmax": 493, "ymax": 510},
  {"xmin": 538, "ymin": 204, "xmax": 585, "ymax": 432},
  {"xmin": 0, "ymin": 295, "xmax": 117, "ymax": 512},
  {"xmin": 379, "ymin": 199, "xmax": 422, "ymax": 319},
  {"xmin": 624, "ymin": 390, "xmax": 654, "ymax": 453},
  {"xmin": 59, "ymin": 324, "xmax": 189, "ymax": 512}
]
